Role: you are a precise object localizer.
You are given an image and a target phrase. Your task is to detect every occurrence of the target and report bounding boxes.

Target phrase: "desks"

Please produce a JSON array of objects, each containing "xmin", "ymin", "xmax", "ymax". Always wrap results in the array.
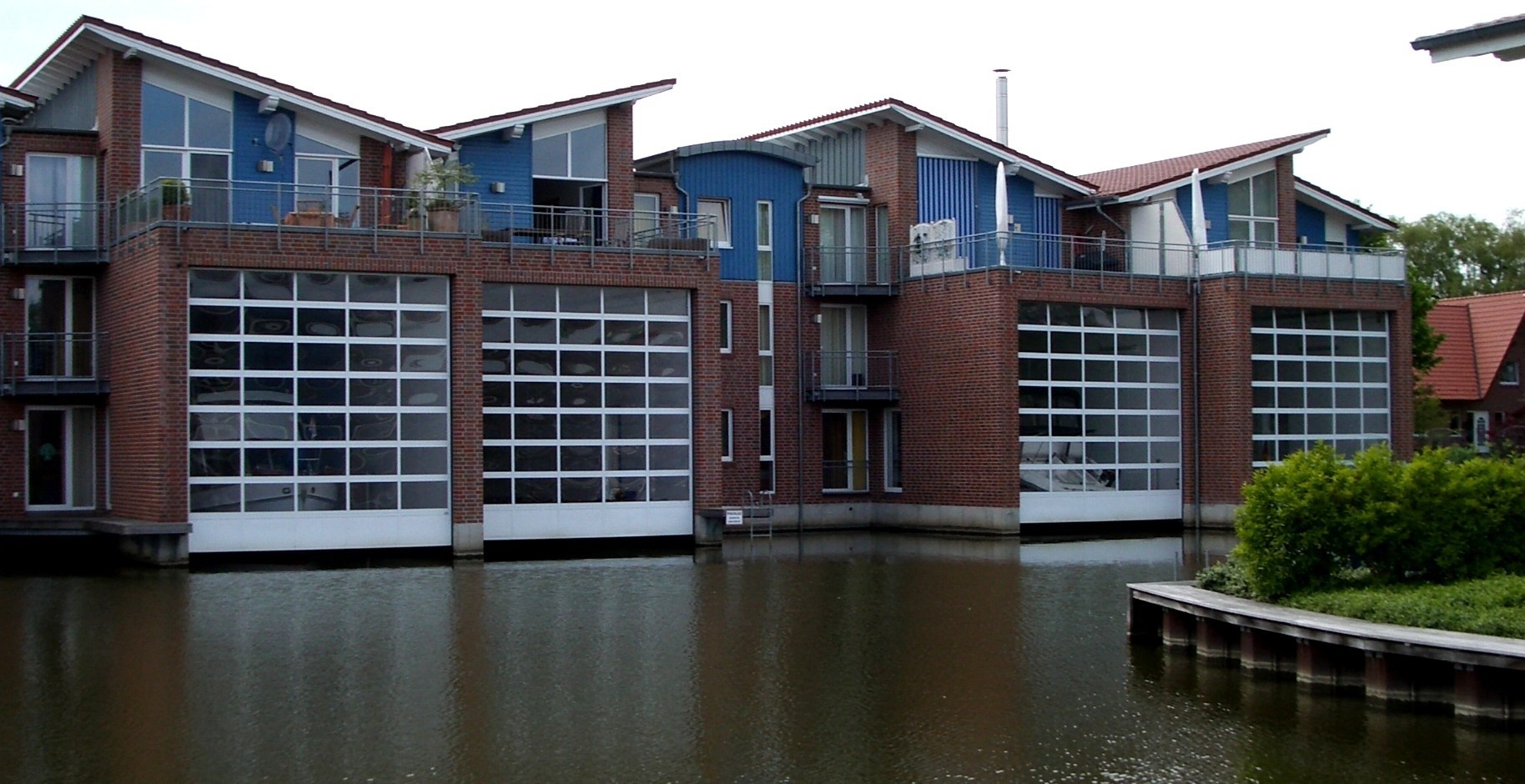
[{"xmin": 285, "ymin": 212, "xmax": 335, "ymax": 227}]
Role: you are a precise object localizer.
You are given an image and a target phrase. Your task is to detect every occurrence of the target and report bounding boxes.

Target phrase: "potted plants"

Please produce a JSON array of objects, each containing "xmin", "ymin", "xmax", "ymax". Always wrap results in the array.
[
  {"xmin": 415, "ymin": 160, "xmax": 480, "ymax": 232},
  {"xmin": 405, "ymin": 192, "xmax": 426, "ymax": 232},
  {"xmin": 163, "ymin": 180, "xmax": 192, "ymax": 221}
]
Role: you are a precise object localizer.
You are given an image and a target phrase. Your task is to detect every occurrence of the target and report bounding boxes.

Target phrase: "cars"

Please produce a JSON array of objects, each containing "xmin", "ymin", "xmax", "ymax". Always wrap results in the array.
[
  {"xmin": 1412, "ymin": 428, "xmax": 1471, "ymax": 456},
  {"xmin": 1495, "ymin": 424, "xmax": 1525, "ymax": 452}
]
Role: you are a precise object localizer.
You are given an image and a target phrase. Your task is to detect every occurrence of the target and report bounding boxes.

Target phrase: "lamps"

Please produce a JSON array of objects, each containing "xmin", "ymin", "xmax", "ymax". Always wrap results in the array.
[
  {"xmin": 808, "ymin": 213, "xmax": 820, "ymax": 225},
  {"xmin": 9, "ymin": 419, "xmax": 26, "ymax": 431},
  {"xmin": 665, "ymin": 205, "xmax": 678, "ymax": 215},
  {"xmin": 258, "ymin": 160, "xmax": 273, "ymax": 172},
  {"xmin": 8, "ymin": 289, "xmax": 24, "ymax": 300},
  {"xmin": 492, "ymin": 182, "xmax": 505, "ymax": 193},
  {"xmin": 8, "ymin": 163, "xmax": 24, "ymax": 177},
  {"xmin": 811, "ymin": 314, "xmax": 822, "ymax": 324},
  {"xmin": 1300, "ymin": 236, "xmax": 1307, "ymax": 244}
]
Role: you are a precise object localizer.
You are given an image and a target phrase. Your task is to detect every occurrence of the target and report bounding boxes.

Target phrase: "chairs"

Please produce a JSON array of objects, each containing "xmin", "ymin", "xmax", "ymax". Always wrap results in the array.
[
  {"xmin": 335, "ymin": 205, "xmax": 360, "ymax": 226},
  {"xmin": 270, "ymin": 204, "xmax": 284, "ymax": 223},
  {"xmin": 910, "ymin": 218, "xmax": 968, "ymax": 276}
]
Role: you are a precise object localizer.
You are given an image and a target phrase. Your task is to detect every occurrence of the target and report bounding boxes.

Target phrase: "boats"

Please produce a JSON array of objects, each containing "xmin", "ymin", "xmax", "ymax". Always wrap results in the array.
[{"xmin": 190, "ymin": 388, "xmax": 340, "ymax": 512}]
